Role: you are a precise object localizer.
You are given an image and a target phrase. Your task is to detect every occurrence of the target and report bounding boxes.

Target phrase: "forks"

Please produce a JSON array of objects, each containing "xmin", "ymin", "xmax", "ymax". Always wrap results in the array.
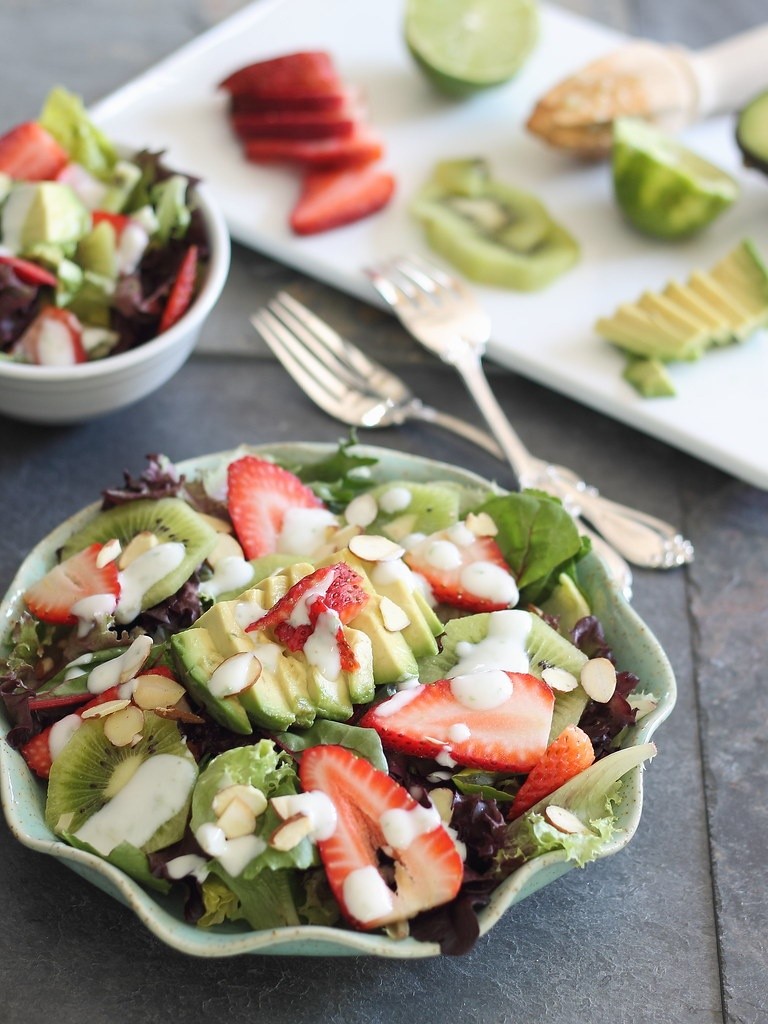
[
  {"xmin": 249, "ymin": 289, "xmax": 699, "ymax": 575},
  {"xmin": 369, "ymin": 237, "xmax": 638, "ymax": 610}
]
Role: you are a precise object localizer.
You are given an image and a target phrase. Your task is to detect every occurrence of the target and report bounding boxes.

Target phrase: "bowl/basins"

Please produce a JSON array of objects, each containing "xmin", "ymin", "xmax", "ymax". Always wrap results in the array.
[
  {"xmin": 1, "ymin": 435, "xmax": 679, "ymax": 961},
  {"xmin": 0, "ymin": 143, "xmax": 231, "ymax": 427}
]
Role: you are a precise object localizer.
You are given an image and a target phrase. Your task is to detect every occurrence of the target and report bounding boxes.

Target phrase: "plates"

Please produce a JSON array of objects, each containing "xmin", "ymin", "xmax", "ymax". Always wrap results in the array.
[{"xmin": 92, "ymin": 0, "xmax": 766, "ymax": 494}]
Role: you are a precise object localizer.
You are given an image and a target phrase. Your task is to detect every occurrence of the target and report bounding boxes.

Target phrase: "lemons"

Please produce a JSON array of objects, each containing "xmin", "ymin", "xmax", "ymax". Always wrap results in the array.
[{"xmin": 405, "ymin": 1, "xmax": 768, "ymax": 396}]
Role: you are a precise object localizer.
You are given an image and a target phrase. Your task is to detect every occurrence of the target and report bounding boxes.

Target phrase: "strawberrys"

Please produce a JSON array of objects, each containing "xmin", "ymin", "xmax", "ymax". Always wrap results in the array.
[
  {"xmin": 0, "ymin": 438, "xmax": 662, "ymax": 954},
  {"xmin": 220, "ymin": 52, "xmax": 395, "ymax": 235}
]
